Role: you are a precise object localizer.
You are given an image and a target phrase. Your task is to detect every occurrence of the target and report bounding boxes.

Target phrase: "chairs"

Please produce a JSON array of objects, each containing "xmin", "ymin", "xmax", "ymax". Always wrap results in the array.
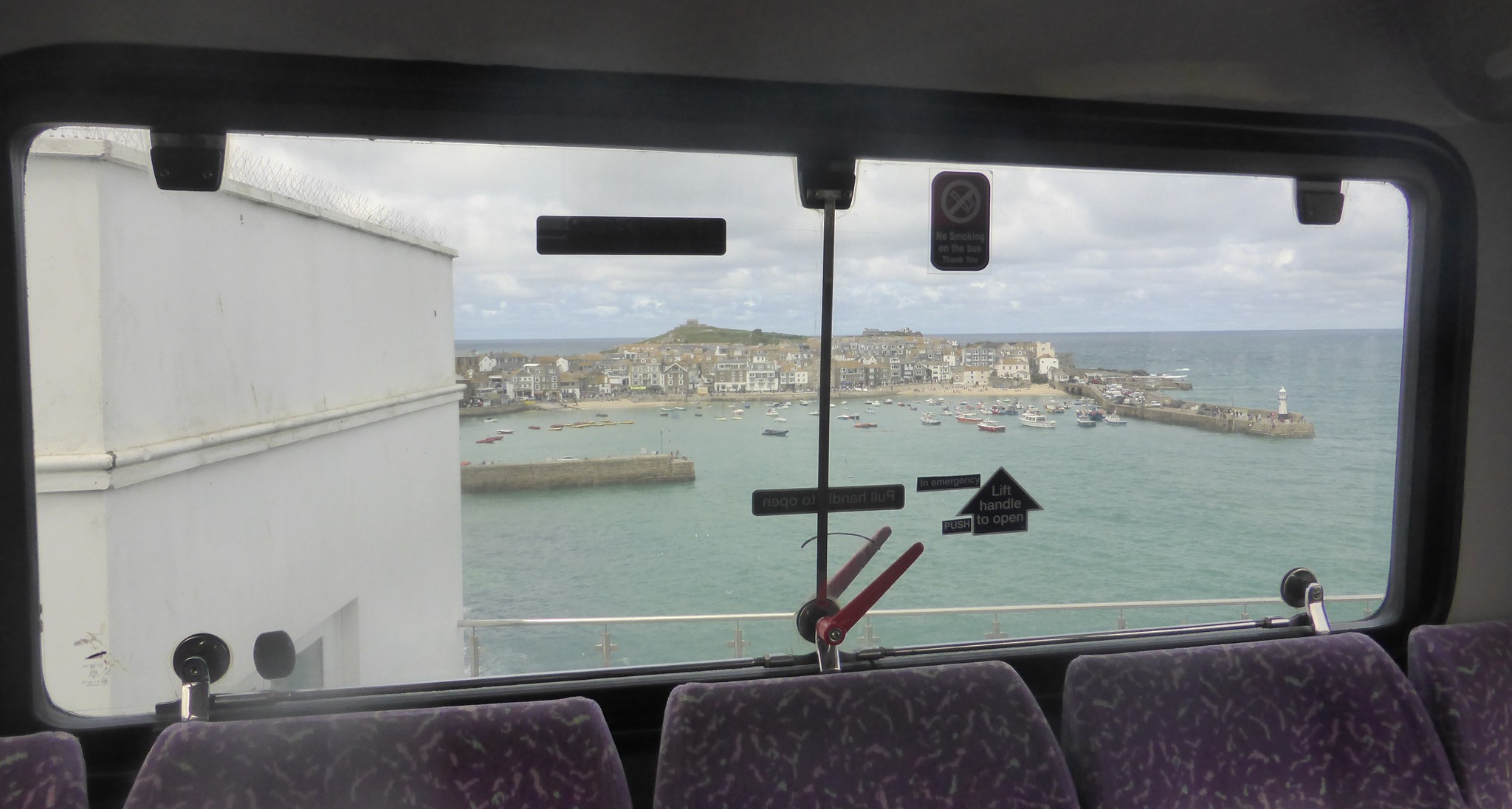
[
  {"xmin": 652, "ymin": 659, "xmax": 1078, "ymax": 809},
  {"xmin": 1405, "ymin": 619, "xmax": 1512, "ymax": 809},
  {"xmin": 1060, "ymin": 630, "xmax": 1464, "ymax": 809},
  {"xmin": 122, "ymin": 696, "xmax": 634, "ymax": 809},
  {"xmin": 0, "ymin": 731, "xmax": 90, "ymax": 809}
]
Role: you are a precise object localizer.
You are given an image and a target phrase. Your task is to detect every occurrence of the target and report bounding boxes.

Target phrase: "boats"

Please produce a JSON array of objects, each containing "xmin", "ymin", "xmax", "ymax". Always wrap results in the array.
[
  {"xmin": 920, "ymin": 416, "xmax": 942, "ymax": 426},
  {"xmin": 809, "ymin": 394, "xmax": 1128, "ymax": 431},
  {"xmin": 977, "ymin": 416, "xmax": 1006, "ymax": 432},
  {"xmin": 528, "ymin": 426, "xmax": 540, "ymax": 429},
  {"xmin": 659, "ymin": 400, "xmax": 712, "ymax": 417},
  {"xmin": 475, "ymin": 429, "xmax": 515, "ymax": 444},
  {"xmin": 954, "ymin": 414, "xmax": 984, "ymax": 424},
  {"xmin": 460, "ymin": 461, "xmax": 471, "ymax": 466},
  {"xmin": 733, "ymin": 409, "xmax": 744, "ymax": 414},
  {"xmin": 1076, "ymin": 414, "xmax": 1096, "ymax": 428},
  {"xmin": 800, "ymin": 400, "xmax": 809, "ymax": 406},
  {"xmin": 713, "ymin": 403, "xmax": 726, "ymax": 420},
  {"xmin": 746, "ymin": 404, "xmax": 750, "ymax": 408},
  {"xmin": 774, "ymin": 417, "xmax": 786, "ymax": 422},
  {"xmin": 1017, "ymin": 407, "xmax": 1056, "ymax": 428},
  {"xmin": 762, "ymin": 429, "xmax": 789, "ymax": 437},
  {"xmin": 727, "ymin": 405, "xmax": 736, "ymax": 409},
  {"xmin": 547, "ymin": 412, "xmax": 635, "ymax": 431},
  {"xmin": 766, "ymin": 400, "xmax": 792, "ymax": 411},
  {"xmin": 766, "ymin": 411, "xmax": 779, "ymax": 416},
  {"xmin": 483, "ymin": 417, "xmax": 498, "ymax": 422}
]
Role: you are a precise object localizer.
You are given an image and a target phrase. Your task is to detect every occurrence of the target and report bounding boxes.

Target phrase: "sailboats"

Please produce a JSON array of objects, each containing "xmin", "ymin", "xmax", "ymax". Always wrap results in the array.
[{"xmin": 730, "ymin": 401, "xmax": 742, "ymax": 420}]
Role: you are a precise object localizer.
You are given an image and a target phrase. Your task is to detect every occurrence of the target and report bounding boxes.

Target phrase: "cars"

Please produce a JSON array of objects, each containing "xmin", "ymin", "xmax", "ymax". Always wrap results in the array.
[
  {"xmin": 557, "ymin": 456, "xmax": 579, "ymax": 460},
  {"xmin": 1067, "ymin": 378, "xmax": 1162, "ymax": 408},
  {"xmin": 547, "ymin": 397, "xmax": 554, "ymax": 402},
  {"xmin": 463, "ymin": 396, "xmax": 483, "ymax": 408}
]
